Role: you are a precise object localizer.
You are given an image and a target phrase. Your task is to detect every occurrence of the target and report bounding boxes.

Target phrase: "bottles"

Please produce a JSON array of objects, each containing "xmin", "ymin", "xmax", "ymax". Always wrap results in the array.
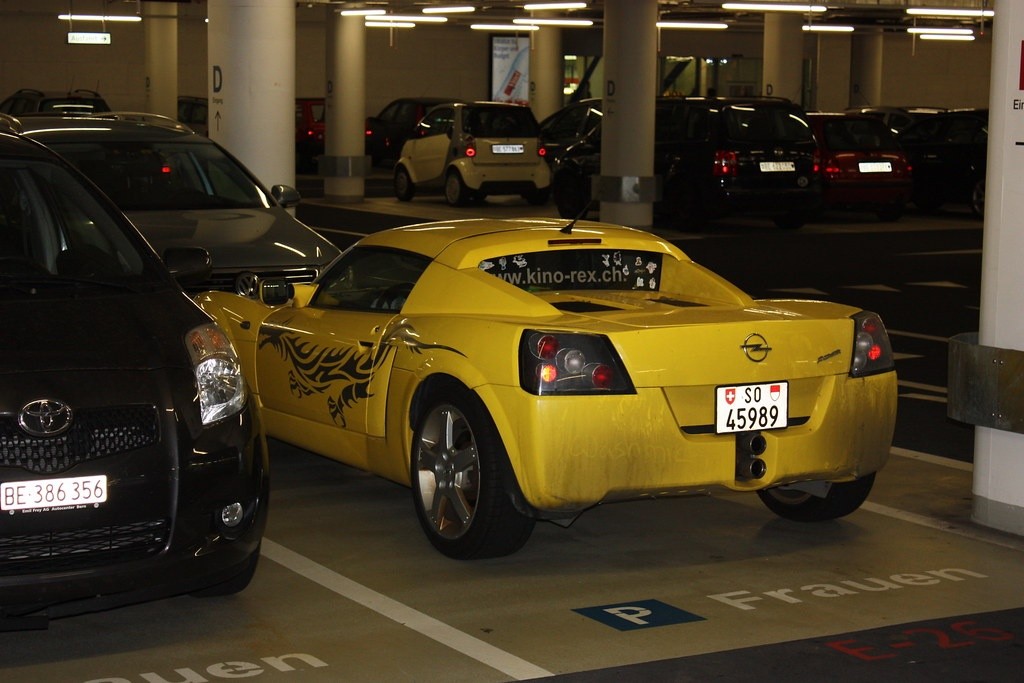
[{"xmin": 496, "ymin": 46, "xmax": 529, "ymax": 102}]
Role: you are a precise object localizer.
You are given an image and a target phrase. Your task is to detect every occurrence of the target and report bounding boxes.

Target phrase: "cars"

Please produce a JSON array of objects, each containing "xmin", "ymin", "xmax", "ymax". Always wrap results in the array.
[
  {"xmin": 291, "ymin": 97, "xmax": 375, "ymax": 165},
  {"xmin": 536, "ymin": 95, "xmax": 603, "ymax": 167},
  {"xmin": 366, "ymin": 99, "xmax": 463, "ymax": 159},
  {"xmin": 176, "ymin": 96, "xmax": 210, "ymax": 137},
  {"xmin": 396, "ymin": 102, "xmax": 552, "ymax": 208},
  {"xmin": 804, "ymin": 105, "xmax": 988, "ymax": 217}
]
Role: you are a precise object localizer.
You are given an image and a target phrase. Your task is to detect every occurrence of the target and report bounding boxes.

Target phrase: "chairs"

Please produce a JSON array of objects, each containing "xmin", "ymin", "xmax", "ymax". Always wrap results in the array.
[
  {"xmin": 860, "ymin": 135, "xmax": 876, "ymax": 147},
  {"xmin": 495, "ymin": 118, "xmax": 513, "ymax": 129},
  {"xmin": 73, "ymin": 159, "xmax": 126, "ymax": 199},
  {"xmin": 949, "ymin": 130, "xmax": 988, "ymax": 144},
  {"xmin": 0, "ymin": 226, "xmax": 31, "ymax": 271},
  {"xmin": 465, "ymin": 111, "xmax": 483, "ymax": 136}
]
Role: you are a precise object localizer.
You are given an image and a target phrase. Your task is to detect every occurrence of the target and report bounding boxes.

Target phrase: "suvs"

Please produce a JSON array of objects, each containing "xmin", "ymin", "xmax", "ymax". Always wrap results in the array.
[
  {"xmin": 0, "ymin": 113, "xmax": 358, "ymax": 320},
  {"xmin": 0, "ymin": 89, "xmax": 112, "ymax": 116},
  {"xmin": 547, "ymin": 96, "xmax": 824, "ymax": 231},
  {"xmin": 0, "ymin": 125, "xmax": 272, "ymax": 601}
]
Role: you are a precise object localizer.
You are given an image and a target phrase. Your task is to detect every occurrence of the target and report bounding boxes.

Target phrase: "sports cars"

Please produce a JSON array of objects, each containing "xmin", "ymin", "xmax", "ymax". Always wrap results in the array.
[{"xmin": 189, "ymin": 218, "xmax": 899, "ymax": 563}]
